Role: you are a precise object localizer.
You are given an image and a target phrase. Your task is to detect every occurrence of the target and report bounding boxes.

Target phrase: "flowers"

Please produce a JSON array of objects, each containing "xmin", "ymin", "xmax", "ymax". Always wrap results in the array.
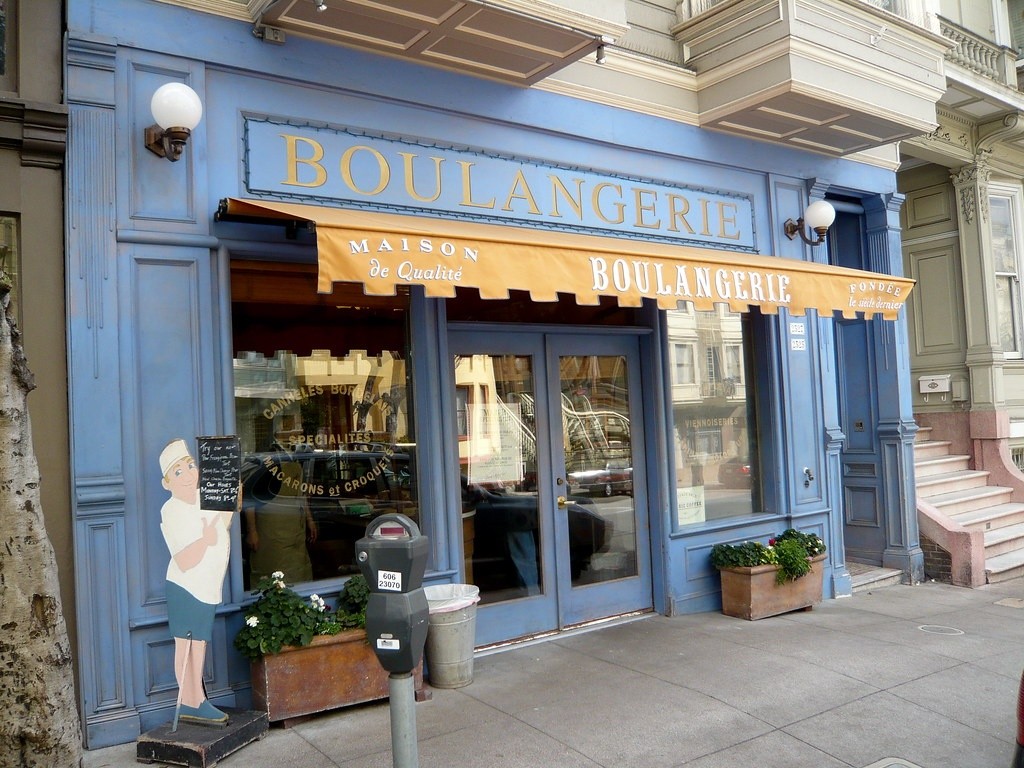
[
  {"xmin": 708, "ymin": 529, "xmax": 827, "ymax": 588},
  {"xmin": 233, "ymin": 571, "xmax": 372, "ymax": 664}
]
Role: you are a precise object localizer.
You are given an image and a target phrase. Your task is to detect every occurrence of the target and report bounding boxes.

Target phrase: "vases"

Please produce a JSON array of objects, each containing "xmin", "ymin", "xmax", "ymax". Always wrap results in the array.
[
  {"xmin": 251, "ymin": 627, "xmax": 424, "ymax": 722},
  {"xmin": 720, "ymin": 553, "xmax": 828, "ymax": 621}
]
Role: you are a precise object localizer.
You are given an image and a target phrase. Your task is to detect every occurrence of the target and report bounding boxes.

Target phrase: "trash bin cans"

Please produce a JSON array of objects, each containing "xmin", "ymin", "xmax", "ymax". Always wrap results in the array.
[{"xmin": 422, "ymin": 583, "xmax": 481, "ymax": 691}]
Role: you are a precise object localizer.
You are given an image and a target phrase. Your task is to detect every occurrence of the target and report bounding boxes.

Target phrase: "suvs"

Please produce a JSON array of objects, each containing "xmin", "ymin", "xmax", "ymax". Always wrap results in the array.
[{"xmin": 234, "ymin": 440, "xmax": 604, "ymax": 595}]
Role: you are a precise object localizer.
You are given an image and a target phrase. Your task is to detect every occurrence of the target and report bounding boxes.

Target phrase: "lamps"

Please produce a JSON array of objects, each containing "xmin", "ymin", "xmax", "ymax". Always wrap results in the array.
[
  {"xmin": 784, "ymin": 201, "xmax": 835, "ymax": 246},
  {"xmin": 145, "ymin": 82, "xmax": 203, "ymax": 163}
]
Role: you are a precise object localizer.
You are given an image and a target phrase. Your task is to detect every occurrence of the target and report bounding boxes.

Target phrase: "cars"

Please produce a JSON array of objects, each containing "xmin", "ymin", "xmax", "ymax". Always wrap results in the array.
[
  {"xmin": 568, "ymin": 466, "xmax": 634, "ymax": 499},
  {"xmin": 718, "ymin": 454, "xmax": 752, "ymax": 490}
]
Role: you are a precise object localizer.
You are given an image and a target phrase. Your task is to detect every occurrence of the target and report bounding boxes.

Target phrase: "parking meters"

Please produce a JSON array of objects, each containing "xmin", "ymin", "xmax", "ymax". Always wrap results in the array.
[{"xmin": 352, "ymin": 514, "xmax": 428, "ymax": 768}]
